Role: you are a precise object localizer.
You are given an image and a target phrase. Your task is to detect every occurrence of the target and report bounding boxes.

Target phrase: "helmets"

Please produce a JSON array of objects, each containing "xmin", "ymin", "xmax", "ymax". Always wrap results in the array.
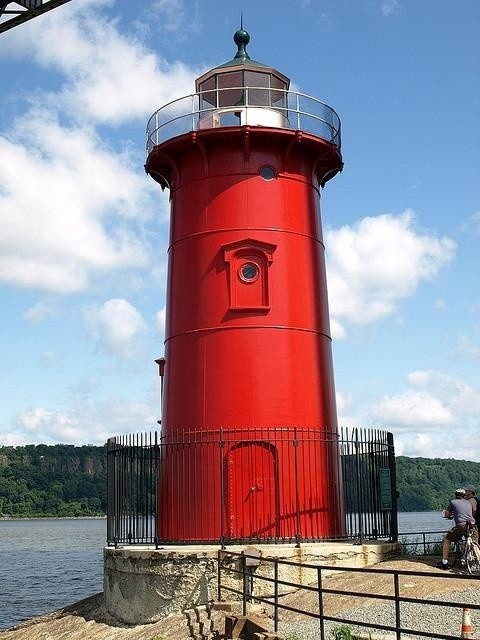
[{"xmin": 455, "ymin": 489, "xmax": 466, "ymax": 494}]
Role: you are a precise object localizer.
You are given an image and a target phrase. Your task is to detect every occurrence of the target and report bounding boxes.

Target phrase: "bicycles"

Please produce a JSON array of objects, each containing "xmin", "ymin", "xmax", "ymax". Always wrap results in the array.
[{"xmin": 444, "ymin": 515, "xmax": 480, "ymax": 576}]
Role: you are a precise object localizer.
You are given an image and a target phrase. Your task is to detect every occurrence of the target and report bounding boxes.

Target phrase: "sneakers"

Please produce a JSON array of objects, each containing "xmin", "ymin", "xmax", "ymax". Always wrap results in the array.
[{"xmin": 437, "ymin": 560, "xmax": 448, "ymax": 570}]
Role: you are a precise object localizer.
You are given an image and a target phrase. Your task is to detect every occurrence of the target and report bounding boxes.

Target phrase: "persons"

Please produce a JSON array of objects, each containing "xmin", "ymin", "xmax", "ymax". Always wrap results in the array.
[
  {"xmin": 437, "ymin": 488, "xmax": 475, "ymax": 569},
  {"xmin": 464, "ymin": 484, "xmax": 480, "ymax": 545}
]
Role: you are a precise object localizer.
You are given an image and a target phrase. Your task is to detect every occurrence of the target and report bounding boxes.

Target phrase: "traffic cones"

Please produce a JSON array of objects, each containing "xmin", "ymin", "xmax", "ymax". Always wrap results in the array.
[{"xmin": 460, "ymin": 608, "xmax": 473, "ymax": 639}]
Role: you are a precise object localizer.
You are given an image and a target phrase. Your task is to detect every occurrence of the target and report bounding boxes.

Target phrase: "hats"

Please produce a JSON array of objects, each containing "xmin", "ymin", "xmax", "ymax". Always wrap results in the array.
[{"xmin": 463, "ymin": 484, "xmax": 476, "ymax": 491}]
